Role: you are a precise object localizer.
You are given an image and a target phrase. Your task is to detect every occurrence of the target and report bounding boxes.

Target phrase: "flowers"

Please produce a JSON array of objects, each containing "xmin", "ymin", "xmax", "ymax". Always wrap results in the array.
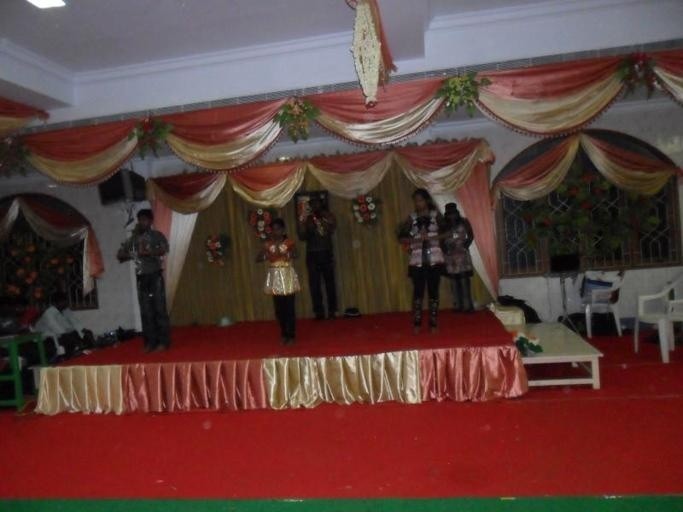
[
  {"xmin": 522, "ymin": 161, "xmax": 662, "ymax": 257},
  {"xmin": 0, "ymin": 233, "xmax": 77, "ymax": 328},
  {"xmin": 619, "ymin": 50, "xmax": 657, "ymax": 102},
  {"xmin": 434, "ymin": 71, "xmax": 491, "ymax": 118}
]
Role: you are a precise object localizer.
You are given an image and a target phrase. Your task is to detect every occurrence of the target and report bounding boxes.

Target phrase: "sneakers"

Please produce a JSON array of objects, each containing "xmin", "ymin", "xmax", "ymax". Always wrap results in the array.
[
  {"xmin": 158, "ymin": 345, "xmax": 168, "ymax": 352},
  {"xmin": 327, "ymin": 314, "xmax": 337, "ymax": 321},
  {"xmin": 465, "ymin": 308, "xmax": 474, "ymax": 313},
  {"xmin": 452, "ymin": 307, "xmax": 462, "ymax": 313},
  {"xmin": 144, "ymin": 345, "xmax": 153, "ymax": 353},
  {"xmin": 280, "ymin": 338, "xmax": 295, "ymax": 346},
  {"xmin": 312, "ymin": 315, "xmax": 325, "ymax": 321}
]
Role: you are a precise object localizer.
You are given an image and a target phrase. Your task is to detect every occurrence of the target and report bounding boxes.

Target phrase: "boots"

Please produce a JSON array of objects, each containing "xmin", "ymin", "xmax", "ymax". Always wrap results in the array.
[
  {"xmin": 428, "ymin": 299, "xmax": 439, "ymax": 334},
  {"xmin": 413, "ymin": 300, "xmax": 422, "ymax": 336}
]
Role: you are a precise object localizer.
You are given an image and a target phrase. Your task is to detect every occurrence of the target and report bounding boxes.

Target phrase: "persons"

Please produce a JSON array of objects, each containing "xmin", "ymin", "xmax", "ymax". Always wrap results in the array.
[
  {"xmin": 394, "ymin": 189, "xmax": 455, "ymax": 333},
  {"xmin": 296, "ymin": 196, "xmax": 341, "ymax": 320},
  {"xmin": 440, "ymin": 203, "xmax": 474, "ymax": 312},
  {"xmin": 117, "ymin": 208, "xmax": 171, "ymax": 355},
  {"xmin": 35, "ymin": 291, "xmax": 87, "ymax": 357},
  {"xmin": 256, "ymin": 218, "xmax": 299, "ymax": 344}
]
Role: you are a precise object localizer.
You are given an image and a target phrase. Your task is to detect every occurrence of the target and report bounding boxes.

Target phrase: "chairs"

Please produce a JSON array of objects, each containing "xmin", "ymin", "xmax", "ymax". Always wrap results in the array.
[{"xmin": 585, "ymin": 274, "xmax": 683, "ymax": 364}]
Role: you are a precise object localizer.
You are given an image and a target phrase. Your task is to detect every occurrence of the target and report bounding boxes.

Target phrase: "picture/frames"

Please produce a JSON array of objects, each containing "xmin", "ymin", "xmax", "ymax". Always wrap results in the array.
[
  {"xmin": 205, "ymin": 232, "xmax": 228, "ymax": 267},
  {"xmin": 298, "ymin": 191, "xmax": 330, "ymax": 237},
  {"xmin": 248, "ymin": 207, "xmax": 296, "ymax": 262},
  {"xmin": 272, "ymin": 96, "xmax": 321, "ymax": 144},
  {"xmin": 129, "ymin": 112, "xmax": 174, "ymax": 160},
  {"xmin": 351, "ymin": 195, "xmax": 376, "ymax": 224},
  {"xmin": 0, "ymin": 137, "xmax": 28, "ymax": 177}
]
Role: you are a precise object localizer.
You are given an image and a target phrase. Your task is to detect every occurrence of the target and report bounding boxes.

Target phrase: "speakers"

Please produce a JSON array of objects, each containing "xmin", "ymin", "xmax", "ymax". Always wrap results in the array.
[
  {"xmin": 551, "ymin": 252, "xmax": 579, "ymax": 272},
  {"xmin": 99, "ymin": 168, "xmax": 146, "ymax": 206}
]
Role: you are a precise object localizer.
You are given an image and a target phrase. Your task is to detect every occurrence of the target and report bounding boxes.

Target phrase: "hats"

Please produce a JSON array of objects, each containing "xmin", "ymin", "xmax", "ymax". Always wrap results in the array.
[
  {"xmin": 344, "ymin": 308, "xmax": 361, "ymax": 320},
  {"xmin": 444, "ymin": 203, "xmax": 458, "ymax": 215},
  {"xmin": 306, "ymin": 192, "xmax": 323, "ymax": 202}
]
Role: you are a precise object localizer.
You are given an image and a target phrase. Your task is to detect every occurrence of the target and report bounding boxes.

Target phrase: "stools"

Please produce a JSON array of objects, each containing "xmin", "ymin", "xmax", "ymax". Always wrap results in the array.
[{"xmin": 0, "ymin": 333, "xmax": 48, "ymax": 412}]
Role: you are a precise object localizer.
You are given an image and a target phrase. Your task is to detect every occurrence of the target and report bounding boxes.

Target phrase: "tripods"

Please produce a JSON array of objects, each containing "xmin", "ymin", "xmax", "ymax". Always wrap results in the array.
[{"xmin": 560, "ymin": 272, "xmax": 579, "ymax": 334}]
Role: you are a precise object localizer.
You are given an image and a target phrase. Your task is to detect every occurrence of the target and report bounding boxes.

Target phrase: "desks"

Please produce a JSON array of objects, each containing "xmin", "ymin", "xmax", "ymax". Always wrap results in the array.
[{"xmin": 503, "ymin": 322, "xmax": 604, "ymax": 390}]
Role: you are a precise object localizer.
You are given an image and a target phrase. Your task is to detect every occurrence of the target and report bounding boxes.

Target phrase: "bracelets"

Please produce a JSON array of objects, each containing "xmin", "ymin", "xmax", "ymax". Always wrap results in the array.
[
  {"xmin": 287, "ymin": 252, "xmax": 291, "ymax": 258},
  {"xmin": 263, "ymin": 255, "xmax": 266, "ymax": 260}
]
